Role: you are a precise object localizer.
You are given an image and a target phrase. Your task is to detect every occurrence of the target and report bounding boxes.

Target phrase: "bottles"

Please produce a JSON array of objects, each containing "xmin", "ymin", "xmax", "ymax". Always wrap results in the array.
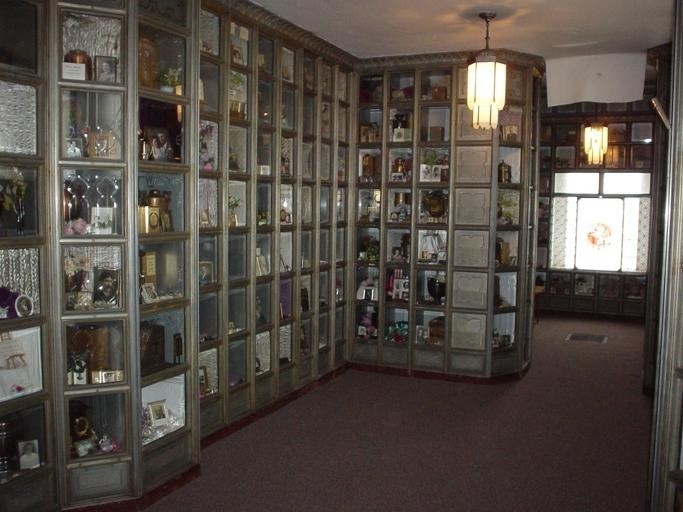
[
  {"xmin": 394, "ymin": 193, "xmax": 405, "ymax": 205},
  {"xmin": 497, "ymin": 159, "xmax": 510, "ymax": 183},
  {"xmin": 64, "ymin": 50, "xmax": 95, "ymax": 81},
  {"xmin": 394, "ymin": 114, "xmax": 406, "ymax": 128},
  {"xmin": 144, "ymin": 189, "xmax": 167, "ymax": 232},
  {"xmin": 394, "ymin": 157, "xmax": 404, "ymax": 172}
]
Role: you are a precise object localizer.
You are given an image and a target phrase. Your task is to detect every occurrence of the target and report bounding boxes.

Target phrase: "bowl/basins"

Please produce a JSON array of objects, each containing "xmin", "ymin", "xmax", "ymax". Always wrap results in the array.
[{"xmin": 229, "ymin": 100, "xmax": 246, "ymax": 121}]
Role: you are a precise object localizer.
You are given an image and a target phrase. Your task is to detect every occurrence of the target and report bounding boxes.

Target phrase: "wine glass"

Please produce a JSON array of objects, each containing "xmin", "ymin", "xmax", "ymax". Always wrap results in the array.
[{"xmin": 427, "ymin": 278, "xmax": 445, "ymax": 305}]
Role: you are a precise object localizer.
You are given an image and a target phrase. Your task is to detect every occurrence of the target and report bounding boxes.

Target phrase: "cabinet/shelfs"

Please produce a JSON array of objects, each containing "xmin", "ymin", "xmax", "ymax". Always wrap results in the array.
[
  {"xmin": 548, "ymin": 268, "xmax": 646, "ymax": 320},
  {"xmin": 527, "ymin": 109, "xmax": 552, "ymax": 315},
  {"xmin": 344, "ymin": 50, "xmax": 547, "ymax": 380},
  {"xmin": 0, "ymin": 1, "xmax": 352, "ymax": 511},
  {"xmin": 553, "ymin": 67, "xmax": 672, "ymax": 177}
]
